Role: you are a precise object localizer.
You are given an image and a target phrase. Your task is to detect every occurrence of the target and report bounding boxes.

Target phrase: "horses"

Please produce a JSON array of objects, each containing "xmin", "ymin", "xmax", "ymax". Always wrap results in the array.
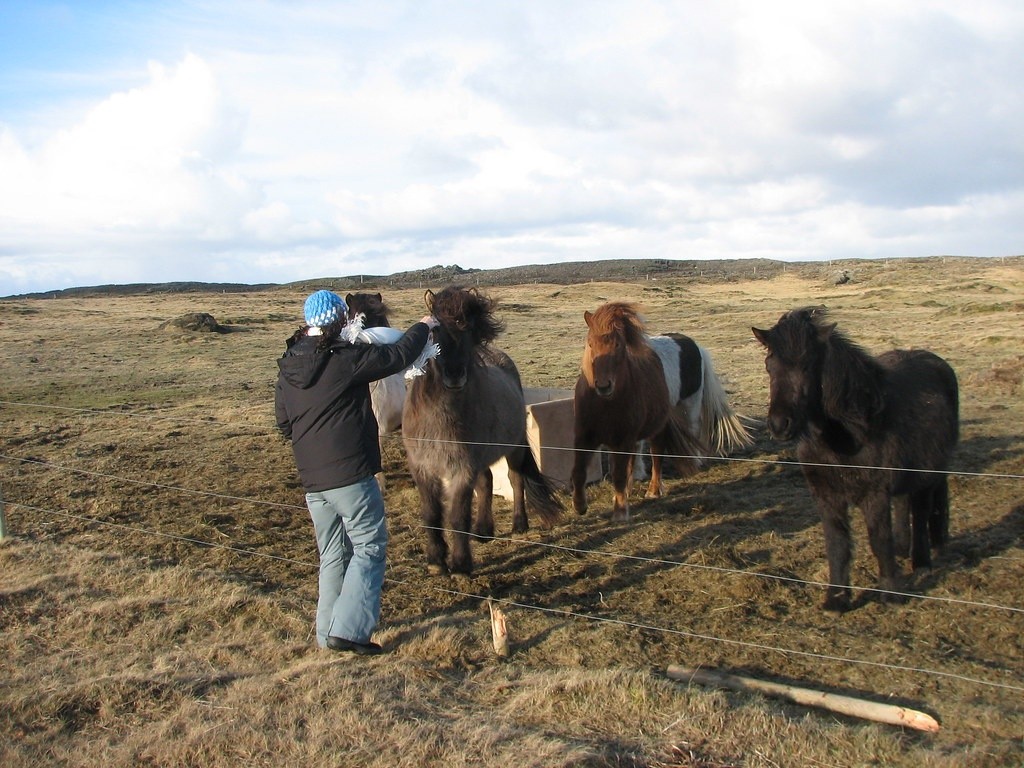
[
  {"xmin": 748, "ymin": 308, "xmax": 960, "ymax": 618},
  {"xmin": 568, "ymin": 300, "xmax": 763, "ymax": 522},
  {"xmin": 399, "ymin": 285, "xmax": 570, "ymax": 583},
  {"xmin": 339, "ymin": 292, "xmax": 443, "ymax": 494}
]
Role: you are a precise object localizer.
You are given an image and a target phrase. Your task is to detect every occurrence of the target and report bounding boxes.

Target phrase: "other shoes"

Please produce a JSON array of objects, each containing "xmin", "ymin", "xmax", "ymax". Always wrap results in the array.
[{"xmin": 328, "ymin": 636, "xmax": 383, "ymax": 656}]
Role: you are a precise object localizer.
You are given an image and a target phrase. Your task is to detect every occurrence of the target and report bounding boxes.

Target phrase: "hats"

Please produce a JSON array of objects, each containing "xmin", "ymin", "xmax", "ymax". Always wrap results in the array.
[{"xmin": 304, "ymin": 290, "xmax": 350, "ymax": 327}]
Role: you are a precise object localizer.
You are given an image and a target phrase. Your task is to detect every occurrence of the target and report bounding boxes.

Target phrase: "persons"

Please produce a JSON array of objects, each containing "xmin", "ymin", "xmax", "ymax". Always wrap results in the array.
[{"xmin": 275, "ymin": 290, "xmax": 441, "ymax": 654}]
